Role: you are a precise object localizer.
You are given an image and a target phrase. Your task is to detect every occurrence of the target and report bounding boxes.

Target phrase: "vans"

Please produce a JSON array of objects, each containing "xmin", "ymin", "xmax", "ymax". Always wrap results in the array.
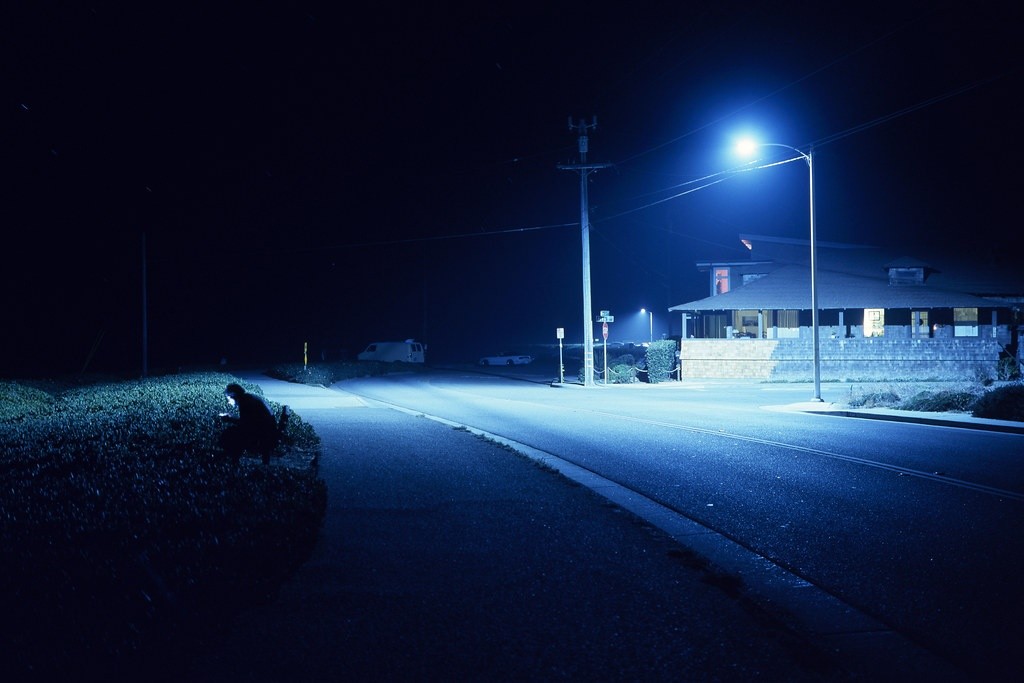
[{"xmin": 356, "ymin": 341, "xmax": 425, "ymax": 365}]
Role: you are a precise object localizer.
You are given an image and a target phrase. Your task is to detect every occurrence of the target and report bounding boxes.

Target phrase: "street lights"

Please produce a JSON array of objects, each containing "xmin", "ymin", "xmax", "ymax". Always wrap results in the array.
[
  {"xmin": 641, "ymin": 308, "xmax": 653, "ymax": 342},
  {"xmin": 735, "ymin": 139, "xmax": 823, "ymax": 402}
]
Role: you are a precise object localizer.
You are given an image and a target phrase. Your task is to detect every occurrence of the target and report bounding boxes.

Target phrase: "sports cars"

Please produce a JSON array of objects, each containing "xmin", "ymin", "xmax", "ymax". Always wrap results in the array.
[{"xmin": 481, "ymin": 350, "xmax": 533, "ymax": 366}]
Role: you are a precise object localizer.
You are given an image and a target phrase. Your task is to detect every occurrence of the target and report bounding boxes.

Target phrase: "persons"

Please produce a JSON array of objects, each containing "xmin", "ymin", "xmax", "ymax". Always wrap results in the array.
[{"xmin": 218, "ymin": 383, "xmax": 277, "ymax": 462}]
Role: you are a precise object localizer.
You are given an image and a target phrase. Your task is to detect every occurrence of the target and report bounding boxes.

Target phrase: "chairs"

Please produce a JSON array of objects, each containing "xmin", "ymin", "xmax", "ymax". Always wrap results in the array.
[{"xmin": 247, "ymin": 405, "xmax": 290, "ymax": 464}]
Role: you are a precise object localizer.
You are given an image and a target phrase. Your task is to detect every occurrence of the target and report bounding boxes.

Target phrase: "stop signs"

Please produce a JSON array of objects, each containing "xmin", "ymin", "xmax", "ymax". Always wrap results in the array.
[{"xmin": 603, "ymin": 323, "xmax": 609, "ymax": 339}]
